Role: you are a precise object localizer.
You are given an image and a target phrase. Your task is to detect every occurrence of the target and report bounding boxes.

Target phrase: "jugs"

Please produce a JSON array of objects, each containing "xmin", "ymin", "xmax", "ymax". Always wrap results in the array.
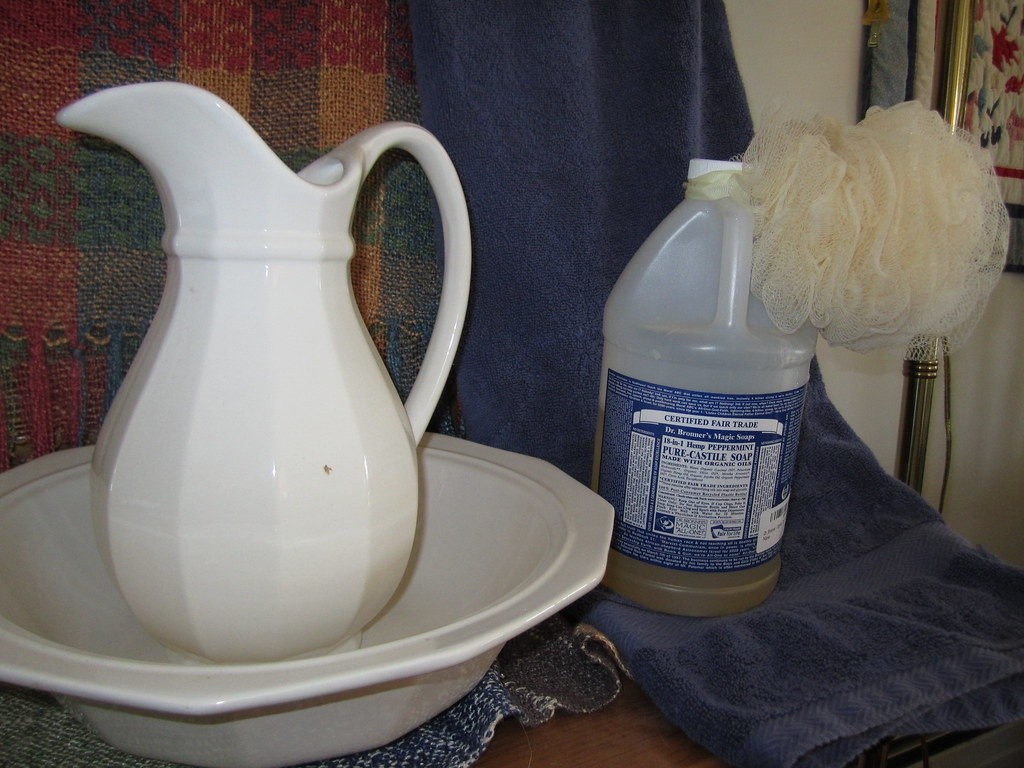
[{"xmin": 56, "ymin": 83, "xmax": 473, "ymax": 672}]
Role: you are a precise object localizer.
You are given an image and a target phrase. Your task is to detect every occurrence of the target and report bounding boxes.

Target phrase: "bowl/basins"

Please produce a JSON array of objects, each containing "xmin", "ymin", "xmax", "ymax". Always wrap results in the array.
[{"xmin": 0, "ymin": 431, "xmax": 615, "ymax": 768}]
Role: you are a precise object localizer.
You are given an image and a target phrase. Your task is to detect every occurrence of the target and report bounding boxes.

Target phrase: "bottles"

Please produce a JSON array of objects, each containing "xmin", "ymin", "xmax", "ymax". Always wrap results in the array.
[{"xmin": 591, "ymin": 157, "xmax": 818, "ymax": 618}]
